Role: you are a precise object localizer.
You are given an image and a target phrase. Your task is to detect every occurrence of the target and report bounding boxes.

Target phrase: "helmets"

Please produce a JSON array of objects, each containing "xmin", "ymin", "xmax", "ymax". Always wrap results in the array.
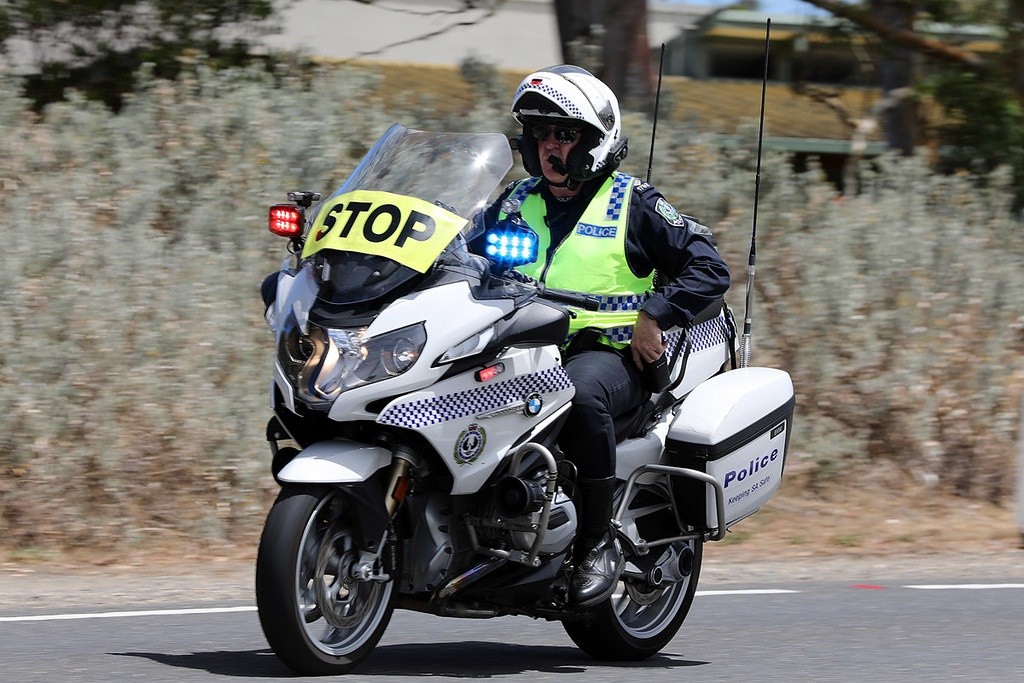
[{"xmin": 511, "ymin": 73, "xmax": 628, "ymax": 181}]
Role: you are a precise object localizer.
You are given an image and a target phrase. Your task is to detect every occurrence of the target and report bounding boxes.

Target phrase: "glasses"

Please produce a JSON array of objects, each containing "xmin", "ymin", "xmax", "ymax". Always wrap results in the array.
[{"xmin": 533, "ymin": 125, "xmax": 582, "ymax": 144}]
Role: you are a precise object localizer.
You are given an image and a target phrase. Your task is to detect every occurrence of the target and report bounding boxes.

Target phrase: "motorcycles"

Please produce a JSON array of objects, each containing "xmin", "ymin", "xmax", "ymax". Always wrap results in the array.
[{"xmin": 254, "ymin": 120, "xmax": 797, "ymax": 676}]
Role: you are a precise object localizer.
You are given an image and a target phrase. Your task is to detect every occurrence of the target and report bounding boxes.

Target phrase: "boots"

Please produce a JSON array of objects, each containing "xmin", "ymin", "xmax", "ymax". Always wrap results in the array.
[{"xmin": 570, "ymin": 473, "xmax": 625, "ymax": 608}]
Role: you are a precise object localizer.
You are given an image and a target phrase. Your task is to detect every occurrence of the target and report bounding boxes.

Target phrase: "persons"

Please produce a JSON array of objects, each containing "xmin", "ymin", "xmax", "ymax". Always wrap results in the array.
[{"xmin": 467, "ymin": 64, "xmax": 732, "ymax": 610}]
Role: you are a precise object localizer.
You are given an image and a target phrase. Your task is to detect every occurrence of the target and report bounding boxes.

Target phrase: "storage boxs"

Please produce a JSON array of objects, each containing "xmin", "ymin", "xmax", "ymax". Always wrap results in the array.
[{"xmin": 665, "ymin": 367, "xmax": 796, "ymax": 531}]
